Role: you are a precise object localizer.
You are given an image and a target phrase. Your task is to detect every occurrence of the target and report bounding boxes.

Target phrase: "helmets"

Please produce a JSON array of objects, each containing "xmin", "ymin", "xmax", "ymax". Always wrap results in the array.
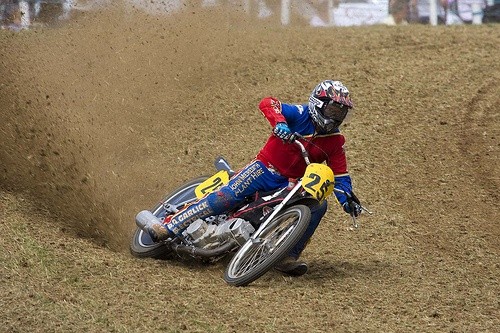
[{"xmin": 307, "ymin": 78, "xmax": 355, "ymax": 131}]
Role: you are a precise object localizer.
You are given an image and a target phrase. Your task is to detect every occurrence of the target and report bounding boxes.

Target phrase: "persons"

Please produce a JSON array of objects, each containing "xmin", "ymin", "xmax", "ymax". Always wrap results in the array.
[{"xmin": 163, "ymin": 80, "xmax": 363, "ymax": 275}]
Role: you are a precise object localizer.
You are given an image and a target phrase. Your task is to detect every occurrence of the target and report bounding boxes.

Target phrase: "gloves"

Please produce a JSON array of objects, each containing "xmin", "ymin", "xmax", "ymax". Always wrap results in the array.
[
  {"xmin": 272, "ymin": 121, "xmax": 295, "ymax": 144},
  {"xmin": 343, "ymin": 200, "xmax": 362, "ymax": 216}
]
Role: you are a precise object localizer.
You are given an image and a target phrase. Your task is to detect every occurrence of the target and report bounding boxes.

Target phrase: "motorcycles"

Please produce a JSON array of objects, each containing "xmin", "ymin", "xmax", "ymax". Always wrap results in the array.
[{"xmin": 129, "ymin": 126, "xmax": 374, "ymax": 287}]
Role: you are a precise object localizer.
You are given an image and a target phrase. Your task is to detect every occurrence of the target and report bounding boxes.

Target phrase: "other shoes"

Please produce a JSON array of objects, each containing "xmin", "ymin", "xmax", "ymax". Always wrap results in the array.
[
  {"xmin": 148, "ymin": 222, "xmax": 167, "ymax": 243},
  {"xmin": 274, "ymin": 255, "xmax": 308, "ymax": 277}
]
